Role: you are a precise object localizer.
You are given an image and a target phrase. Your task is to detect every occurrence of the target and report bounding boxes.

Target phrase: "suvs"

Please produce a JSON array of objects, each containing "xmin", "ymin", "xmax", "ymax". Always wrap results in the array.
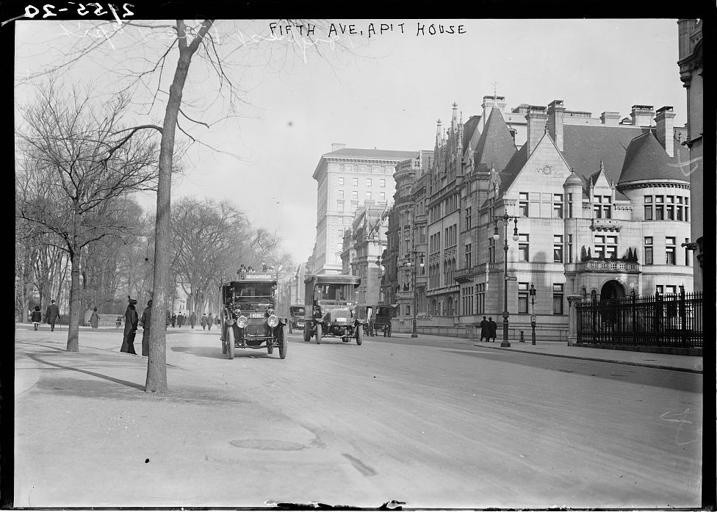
[
  {"xmin": 303, "ymin": 273, "xmax": 363, "ymax": 345},
  {"xmin": 355, "ymin": 305, "xmax": 395, "ymax": 337},
  {"xmin": 289, "ymin": 304, "xmax": 305, "ymax": 335},
  {"xmin": 220, "ymin": 272, "xmax": 287, "ymax": 359}
]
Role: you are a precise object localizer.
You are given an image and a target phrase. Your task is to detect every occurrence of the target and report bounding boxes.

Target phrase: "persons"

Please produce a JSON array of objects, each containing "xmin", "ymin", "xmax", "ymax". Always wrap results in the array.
[
  {"xmin": 479, "ymin": 316, "xmax": 490, "ymax": 342},
  {"xmin": 256, "ymin": 292, "xmax": 271, "ymax": 303},
  {"xmin": 171, "ymin": 312, "xmax": 219, "ymax": 331},
  {"xmin": 120, "ymin": 300, "xmax": 138, "ymax": 355},
  {"xmin": 487, "ymin": 317, "xmax": 497, "ymax": 342},
  {"xmin": 45, "ymin": 300, "xmax": 60, "ymax": 331},
  {"xmin": 31, "ymin": 306, "xmax": 42, "ymax": 331},
  {"xmin": 89, "ymin": 308, "xmax": 100, "ymax": 329},
  {"xmin": 236, "ymin": 264, "xmax": 247, "ymax": 279},
  {"xmin": 141, "ymin": 300, "xmax": 152, "ymax": 356},
  {"xmin": 247, "ymin": 267, "xmax": 256, "ymax": 274}
]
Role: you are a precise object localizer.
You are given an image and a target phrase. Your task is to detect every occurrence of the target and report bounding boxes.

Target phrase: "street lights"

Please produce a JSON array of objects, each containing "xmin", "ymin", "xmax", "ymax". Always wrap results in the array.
[
  {"xmin": 491, "ymin": 208, "xmax": 520, "ymax": 348},
  {"xmin": 529, "ymin": 281, "xmax": 536, "ymax": 345},
  {"xmin": 405, "ymin": 245, "xmax": 425, "ymax": 338},
  {"xmin": 629, "ymin": 281, "xmax": 635, "ymax": 296}
]
